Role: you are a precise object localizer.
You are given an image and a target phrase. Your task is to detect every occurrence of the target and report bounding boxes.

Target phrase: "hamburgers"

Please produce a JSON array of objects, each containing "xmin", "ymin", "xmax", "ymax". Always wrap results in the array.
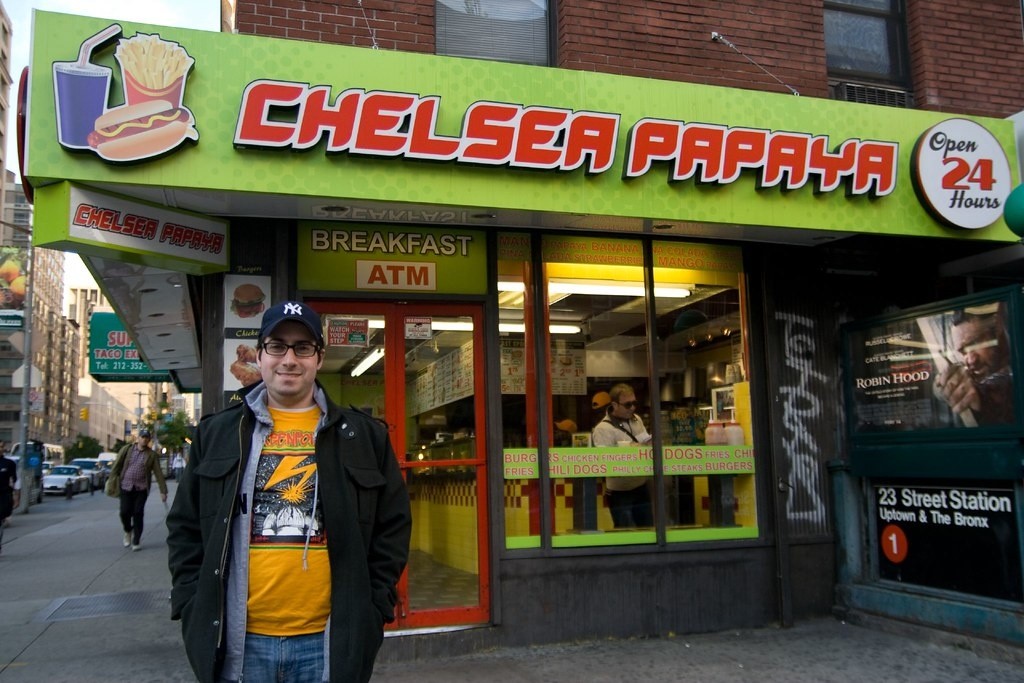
[{"xmin": 230, "ymin": 284, "xmax": 267, "ymax": 318}]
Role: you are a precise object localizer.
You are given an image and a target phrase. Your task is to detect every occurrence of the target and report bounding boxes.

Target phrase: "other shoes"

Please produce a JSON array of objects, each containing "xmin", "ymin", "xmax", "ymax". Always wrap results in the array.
[
  {"xmin": 132, "ymin": 541, "xmax": 142, "ymax": 551},
  {"xmin": 123, "ymin": 532, "xmax": 131, "ymax": 547}
]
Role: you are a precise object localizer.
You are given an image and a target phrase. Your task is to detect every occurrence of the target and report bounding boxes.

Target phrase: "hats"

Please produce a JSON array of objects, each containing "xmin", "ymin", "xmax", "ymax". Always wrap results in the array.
[
  {"xmin": 591, "ymin": 392, "xmax": 611, "ymax": 409},
  {"xmin": 555, "ymin": 420, "xmax": 577, "ymax": 434},
  {"xmin": 258, "ymin": 301, "xmax": 323, "ymax": 348},
  {"xmin": 139, "ymin": 430, "xmax": 151, "ymax": 436}
]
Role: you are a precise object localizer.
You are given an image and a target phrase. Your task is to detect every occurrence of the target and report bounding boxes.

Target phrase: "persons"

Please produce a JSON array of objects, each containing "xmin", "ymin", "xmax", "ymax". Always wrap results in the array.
[
  {"xmin": 932, "ymin": 313, "xmax": 1015, "ymax": 428},
  {"xmin": 105, "ymin": 430, "xmax": 168, "ymax": 551},
  {"xmin": 0, "ymin": 440, "xmax": 22, "ymax": 554},
  {"xmin": 172, "ymin": 452, "xmax": 187, "ymax": 482},
  {"xmin": 592, "ymin": 383, "xmax": 654, "ymax": 528},
  {"xmin": 165, "ymin": 302, "xmax": 413, "ymax": 683}
]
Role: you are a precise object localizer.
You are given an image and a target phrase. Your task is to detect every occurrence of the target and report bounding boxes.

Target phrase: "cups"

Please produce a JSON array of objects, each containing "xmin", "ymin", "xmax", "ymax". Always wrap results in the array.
[
  {"xmin": 162, "ymin": 499, "xmax": 169, "ymax": 511},
  {"xmin": 724, "ymin": 364, "xmax": 740, "ymax": 384}
]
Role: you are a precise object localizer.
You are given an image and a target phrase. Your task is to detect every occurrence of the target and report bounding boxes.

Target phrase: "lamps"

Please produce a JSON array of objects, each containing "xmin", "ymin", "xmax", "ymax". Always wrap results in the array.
[
  {"xmin": 432, "ymin": 321, "xmax": 585, "ymax": 334},
  {"xmin": 720, "ymin": 325, "xmax": 731, "ymax": 335},
  {"xmin": 705, "ymin": 333, "xmax": 714, "ymax": 341},
  {"xmin": 687, "ymin": 337, "xmax": 698, "ymax": 347},
  {"xmin": 350, "ymin": 344, "xmax": 385, "ymax": 379},
  {"xmin": 497, "ymin": 278, "xmax": 697, "ymax": 299}
]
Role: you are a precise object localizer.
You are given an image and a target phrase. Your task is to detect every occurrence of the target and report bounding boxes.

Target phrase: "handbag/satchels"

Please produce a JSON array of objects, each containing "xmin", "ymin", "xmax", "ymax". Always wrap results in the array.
[{"xmin": 105, "ymin": 475, "xmax": 120, "ymax": 498}]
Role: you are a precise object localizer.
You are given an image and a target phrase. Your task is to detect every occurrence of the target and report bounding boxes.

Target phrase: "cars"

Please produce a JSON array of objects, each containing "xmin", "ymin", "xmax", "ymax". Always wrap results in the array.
[
  {"xmin": 67, "ymin": 457, "xmax": 107, "ymax": 489},
  {"xmin": 42, "ymin": 464, "xmax": 91, "ymax": 497}
]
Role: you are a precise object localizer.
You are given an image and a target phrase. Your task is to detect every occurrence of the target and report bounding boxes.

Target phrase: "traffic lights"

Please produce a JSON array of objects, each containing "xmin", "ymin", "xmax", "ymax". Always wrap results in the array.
[{"xmin": 79, "ymin": 407, "xmax": 88, "ymax": 421}]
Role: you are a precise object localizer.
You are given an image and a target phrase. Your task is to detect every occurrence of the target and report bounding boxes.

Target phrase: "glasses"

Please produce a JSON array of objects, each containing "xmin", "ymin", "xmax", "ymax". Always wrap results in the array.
[
  {"xmin": 260, "ymin": 342, "xmax": 322, "ymax": 357},
  {"xmin": 618, "ymin": 401, "xmax": 641, "ymax": 409}
]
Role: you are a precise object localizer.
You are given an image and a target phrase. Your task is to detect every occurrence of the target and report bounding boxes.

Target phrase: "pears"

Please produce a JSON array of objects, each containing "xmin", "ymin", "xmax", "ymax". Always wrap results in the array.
[{"xmin": 0, "ymin": 255, "xmax": 27, "ymax": 301}]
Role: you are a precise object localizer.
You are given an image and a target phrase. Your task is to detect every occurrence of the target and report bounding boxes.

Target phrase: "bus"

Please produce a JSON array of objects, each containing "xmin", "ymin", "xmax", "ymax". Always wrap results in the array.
[
  {"xmin": 8, "ymin": 442, "xmax": 65, "ymax": 479},
  {"xmin": 98, "ymin": 452, "xmax": 119, "ymax": 480}
]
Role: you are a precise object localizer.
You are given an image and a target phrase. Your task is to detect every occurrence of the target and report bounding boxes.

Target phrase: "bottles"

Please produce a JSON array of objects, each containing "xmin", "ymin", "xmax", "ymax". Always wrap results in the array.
[
  {"xmin": 724, "ymin": 422, "xmax": 745, "ymax": 446},
  {"xmin": 705, "ymin": 422, "xmax": 727, "ymax": 447}
]
